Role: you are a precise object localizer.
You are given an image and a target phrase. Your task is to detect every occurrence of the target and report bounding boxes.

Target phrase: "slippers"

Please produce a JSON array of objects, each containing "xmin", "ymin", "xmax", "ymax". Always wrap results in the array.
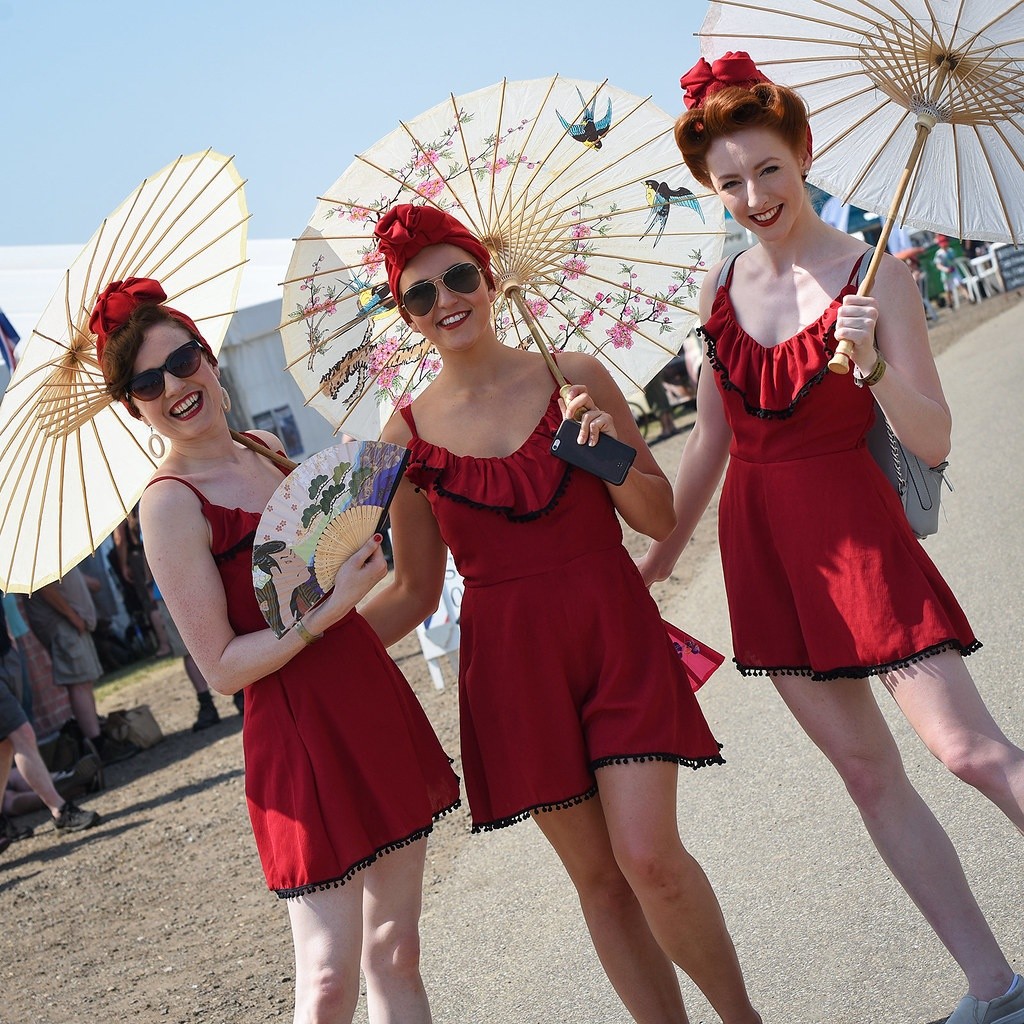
[{"xmin": 148, "ymin": 651, "xmax": 172, "ymax": 662}]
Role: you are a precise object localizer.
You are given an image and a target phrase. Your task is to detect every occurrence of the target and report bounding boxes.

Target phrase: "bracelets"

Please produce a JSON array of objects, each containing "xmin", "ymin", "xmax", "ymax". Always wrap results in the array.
[{"xmin": 853, "ymin": 346, "xmax": 886, "ymax": 388}]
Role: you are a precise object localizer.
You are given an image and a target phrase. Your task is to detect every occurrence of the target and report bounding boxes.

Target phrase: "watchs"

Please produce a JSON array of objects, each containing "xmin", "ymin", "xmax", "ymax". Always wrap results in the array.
[{"xmin": 296, "ymin": 619, "xmax": 324, "ymax": 645}]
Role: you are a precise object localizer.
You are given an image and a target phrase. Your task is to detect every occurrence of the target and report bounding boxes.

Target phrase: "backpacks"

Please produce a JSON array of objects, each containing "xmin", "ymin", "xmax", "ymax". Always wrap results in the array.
[{"xmin": 715, "ymin": 245, "xmax": 952, "ymax": 540}]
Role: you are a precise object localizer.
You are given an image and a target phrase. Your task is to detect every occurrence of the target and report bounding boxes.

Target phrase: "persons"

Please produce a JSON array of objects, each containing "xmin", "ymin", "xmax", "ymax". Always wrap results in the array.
[
  {"xmin": 636, "ymin": 80, "xmax": 1024, "ymax": 1024},
  {"xmin": 905, "ymin": 256, "xmax": 923, "ymax": 286},
  {"xmin": 143, "ymin": 551, "xmax": 245, "ymax": 733},
  {"xmin": 21, "ymin": 565, "xmax": 138, "ymax": 769},
  {"xmin": 963, "ymin": 240, "xmax": 986, "ymax": 300},
  {"xmin": 103, "ymin": 301, "xmax": 461, "ymax": 1024},
  {"xmin": 353, "ymin": 205, "xmax": 764, "ymax": 1024},
  {"xmin": 116, "ymin": 499, "xmax": 174, "ymax": 660},
  {"xmin": 933, "ymin": 234, "xmax": 974, "ymax": 309},
  {"xmin": 0, "ymin": 590, "xmax": 101, "ymax": 843},
  {"xmin": 644, "ymin": 373, "xmax": 683, "ymax": 438}
]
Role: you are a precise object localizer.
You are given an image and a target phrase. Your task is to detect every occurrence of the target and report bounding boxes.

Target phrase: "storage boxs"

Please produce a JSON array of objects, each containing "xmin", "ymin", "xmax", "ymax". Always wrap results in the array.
[{"xmin": 104, "ymin": 703, "xmax": 164, "ymax": 749}]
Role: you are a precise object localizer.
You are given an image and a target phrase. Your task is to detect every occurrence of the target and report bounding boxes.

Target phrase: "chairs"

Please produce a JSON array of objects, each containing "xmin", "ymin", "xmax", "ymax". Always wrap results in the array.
[
  {"xmin": 950, "ymin": 253, "xmax": 1003, "ymax": 311},
  {"xmin": 916, "ymin": 271, "xmax": 938, "ymax": 321}
]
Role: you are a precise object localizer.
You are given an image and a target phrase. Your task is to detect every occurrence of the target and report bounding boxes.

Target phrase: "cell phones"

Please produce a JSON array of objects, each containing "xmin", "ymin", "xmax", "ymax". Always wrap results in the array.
[{"xmin": 550, "ymin": 419, "xmax": 637, "ymax": 486}]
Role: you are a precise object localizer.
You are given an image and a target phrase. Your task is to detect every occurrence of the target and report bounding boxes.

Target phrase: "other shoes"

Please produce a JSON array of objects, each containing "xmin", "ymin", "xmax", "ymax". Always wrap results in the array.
[
  {"xmin": 192, "ymin": 701, "xmax": 220, "ymax": 732},
  {"xmin": 82, "ymin": 738, "xmax": 105, "ymax": 792},
  {"xmin": 53, "ymin": 754, "xmax": 100, "ymax": 792},
  {"xmin": 233, "ymin": 693, "xmax": 244, "ymax": 714},
  {"xmin": 657, "ymin": 431, "xmax": 674, "ymax": 441},
  {"xmin": 91, "ymin": 733, "xmax": 137, "ymax": 764},
  {"xmin": 671, "ymin": 427, "xmax": 680, "ymax": 436}
]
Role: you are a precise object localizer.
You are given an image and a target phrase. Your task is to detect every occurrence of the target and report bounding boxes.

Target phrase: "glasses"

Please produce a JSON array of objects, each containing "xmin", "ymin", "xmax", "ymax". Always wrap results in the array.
[
  {"xmin": 400, "ymin": 262, "xmax": 481, "ymax": 318},
  {"xmin": 123, "ymin": 340, "xmax": 207, "ymax": 402}
]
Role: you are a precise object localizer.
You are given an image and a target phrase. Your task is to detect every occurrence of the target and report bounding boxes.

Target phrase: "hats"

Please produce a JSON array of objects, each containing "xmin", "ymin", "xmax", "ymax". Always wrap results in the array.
[{"xmin": 937, "ymin": 235, "xmax": 948, "ymax": 247}]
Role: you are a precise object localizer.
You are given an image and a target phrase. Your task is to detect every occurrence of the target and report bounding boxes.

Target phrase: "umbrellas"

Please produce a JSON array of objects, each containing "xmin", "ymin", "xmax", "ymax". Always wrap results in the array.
[
  {"xmin": 692, "ymin": 1, "xmax": 1024, "ymax": 375},
  {"xmin": 271, "ymin": 73, "xmax": 733, "ymax": 442},
  {"xmin": 0, "ymin": 148, "xmax": 301, "ymax": 592}
]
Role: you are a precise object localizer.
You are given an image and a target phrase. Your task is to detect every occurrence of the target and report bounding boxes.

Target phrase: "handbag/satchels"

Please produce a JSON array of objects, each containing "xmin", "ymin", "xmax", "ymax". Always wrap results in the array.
[{"xmin": 105, "ymin": 705, "xmax": 163, "ymax": 750}]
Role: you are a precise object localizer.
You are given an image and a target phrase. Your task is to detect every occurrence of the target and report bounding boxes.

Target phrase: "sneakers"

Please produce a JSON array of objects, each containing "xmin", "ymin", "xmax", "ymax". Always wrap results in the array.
[
  {"xmin": 0, "ymin": 814, "xmax": 33, "ymax": 842},
  {"xmin": 52, "ymin": 801, "xmax": 100, "ymax": 833},
  {"xmin": 944, "ymin": 974, "xmax": 1024, "ymax": 1024}
]
